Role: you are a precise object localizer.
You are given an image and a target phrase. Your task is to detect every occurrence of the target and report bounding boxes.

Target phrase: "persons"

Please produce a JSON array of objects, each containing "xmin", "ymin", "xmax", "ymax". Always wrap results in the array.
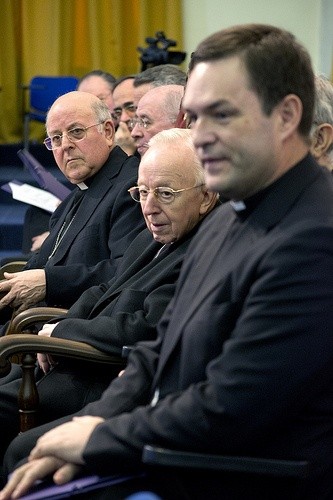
[
  {"xmin": 1, "ymin": 65, "xmax": 188, "ymax": 262},
  {"xmin": 1, "ymin": 25, "xmax": 333, "ymax": 500},
  {"xmin": 0, "ymin": 129, "xmax": 224, "ymax": 486},
  {"xmin": 1, "ymin": 92, "xmax": 153, "ymax": 368},
  {"xmin": 309, "ymin": 72, "xmax": 333, "ymax": 174}
]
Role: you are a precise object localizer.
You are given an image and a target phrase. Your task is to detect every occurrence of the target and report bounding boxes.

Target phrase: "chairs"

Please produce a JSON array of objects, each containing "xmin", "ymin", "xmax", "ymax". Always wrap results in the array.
[
  {"xmin": 0, "ymin": 256, "xmax": 333, "ymax": 500},
  {"xmin": 20, "ymin": 75, "xmax": 83, "ymax": 155}
]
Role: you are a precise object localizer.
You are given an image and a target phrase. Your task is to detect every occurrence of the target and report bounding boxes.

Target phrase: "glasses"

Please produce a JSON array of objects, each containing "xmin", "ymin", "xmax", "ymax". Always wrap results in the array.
[
  {"xmin": 43, "ymin": 121, "xmax": 105, "ymax": 150},
  {"xmin": 111, "ymin": 105, "xmax": 138, "ymax": 122},
  {"xmin": 128, "ymin": 184, "xmax": 204, "ymax": 203},
  {"xmin": 130, "ymin": 117, "xmax": 177, "ymax": 129}
]
